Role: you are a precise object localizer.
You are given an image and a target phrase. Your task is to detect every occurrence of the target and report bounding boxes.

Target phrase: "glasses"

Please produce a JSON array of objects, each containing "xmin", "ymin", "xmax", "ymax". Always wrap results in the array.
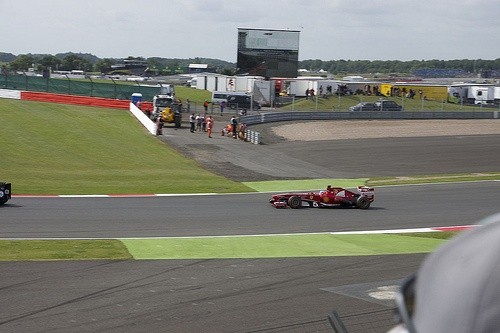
[{"xmin": 395, "ymin": 275, "xmax": 417, "ymax": 333}]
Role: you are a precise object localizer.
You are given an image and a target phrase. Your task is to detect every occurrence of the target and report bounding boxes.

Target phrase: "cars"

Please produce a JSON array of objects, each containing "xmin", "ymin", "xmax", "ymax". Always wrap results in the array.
[
  {"xmin": 349, "ymin": 102, "xmax": 380, "ymax": 112},
  {"xmin": 0, "ymin": 180, "xmax": 12, "ymax": 207}
]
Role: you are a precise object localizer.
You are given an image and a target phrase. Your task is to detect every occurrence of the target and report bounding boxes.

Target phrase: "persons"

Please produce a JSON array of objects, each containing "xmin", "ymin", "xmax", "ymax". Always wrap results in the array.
[
  {"xmin": 220, "ymin": 101, "xmax": 225, "ymax": 116},
  {"xmin": 189, "ymin": 112, "xmax": 196, "ymax": 133},
  {"xmin": 326, "ymin": 85, "xmax": 332, "ymax": 95},
  {"xmin": 319, "ymin": 85, "xmax": 322, "ymax": 94},
  {"xmin": 239, "ymin": 108, "xmax": 246, "ymax": 116},
  {"xmin": 240, "ymin": 122, "xmax": 247, "ymax": 136},
  {"xmin": 196, "ymin": 113, "xmax": 214, "ymax": 132},
  {"xmin": 222, "ymin": 124, "xmax": 231, "ymax": 136},
  {"xmin": 305, "ymin": 88, "xmax": 314, "ymax": 100},
  {"xmin": 206, "ymin": 120, "xmax": 213, "ymax": 138},
  {"xmin": 204, "ymin": 100, "xmax": 208, "ymax": 112},
  {"xmin": 186, "ymin": 99, "xmax": 190, "ymax": 113},
  {"xmin": 230, "ymin": 115, "xmax": 237, "ymax": 132},
  {"xmin": 397, "ymin": 215, "xmax": 500, "ymax": 333},
  {"xmin": 136, "ymin": 100, "xmax": 141, "ymax": 110},
  {"xmin": 145, "ymin": 108, "xmax": 164, "ymax": 135},
  {"xmin": 391, "ymin": 86, "xmax": 414, "ymax": 99},
  {"xmin": 319, "ymin": 184, "xmax": 335, "ymax": 198},
  {"xmin": 367, "ymin": 85, "xmax": 379, "ymax": 93}
]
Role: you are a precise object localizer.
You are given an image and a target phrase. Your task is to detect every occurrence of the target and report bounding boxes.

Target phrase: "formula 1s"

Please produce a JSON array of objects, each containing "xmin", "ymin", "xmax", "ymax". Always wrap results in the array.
[{"xmin": 269, "ymin": 185, "xmax": 375, "ymax": 210}]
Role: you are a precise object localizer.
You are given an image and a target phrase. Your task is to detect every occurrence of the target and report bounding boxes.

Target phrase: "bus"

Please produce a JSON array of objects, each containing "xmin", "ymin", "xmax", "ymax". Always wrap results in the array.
[
  {"xmin": 211, "ymin": 91, "xmax": 252, "ymax": 105},
  {"xmin": 152, "ymin": 94, "xmax": 176, "ymax": 123}
]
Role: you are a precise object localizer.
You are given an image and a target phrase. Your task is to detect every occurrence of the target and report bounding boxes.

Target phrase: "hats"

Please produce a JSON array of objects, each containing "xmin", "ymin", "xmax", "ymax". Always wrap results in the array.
[{"xmin": 389, "ymin": 215, "xmax": 499, "ymax": 333}]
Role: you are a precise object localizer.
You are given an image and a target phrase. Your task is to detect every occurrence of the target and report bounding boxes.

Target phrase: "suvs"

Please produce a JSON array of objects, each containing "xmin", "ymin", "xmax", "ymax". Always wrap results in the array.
[
  {"xmin": 226, "ymin": 94, "xmax": 262, "ymax": 110},
  {"xmin": 374, "ymin": 100, "xmax": 404, "ymax": 111}
]
